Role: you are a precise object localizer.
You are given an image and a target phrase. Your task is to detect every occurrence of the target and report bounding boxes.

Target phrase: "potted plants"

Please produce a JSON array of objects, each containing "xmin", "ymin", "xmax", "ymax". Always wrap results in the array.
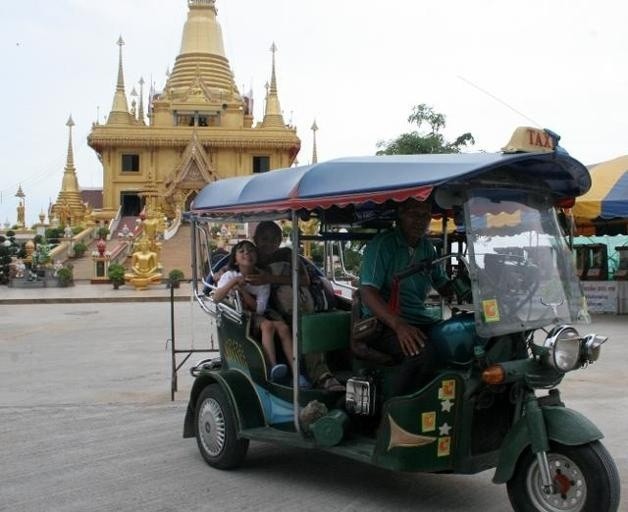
[
  {"xmin": 108, "ymin": 263, "xmax": 126, "ymax": 289},
  {"xmin": 73, "ymin": 244, "xmax": 88, "ymax": 257},
  {"xmin": 169, "ymin": 269, "xmax": 185, "ymax": 288},
  {"xmin": 97, "ymin": 228, "xmax": 111, "ymax": 239},
  {"xmin": 58, "ymin": 268, "xmax": 73, "ymax": 287}
]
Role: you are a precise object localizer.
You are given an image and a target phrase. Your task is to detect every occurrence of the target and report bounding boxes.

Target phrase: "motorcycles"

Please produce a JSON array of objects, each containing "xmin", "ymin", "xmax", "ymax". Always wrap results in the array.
[{"xmin": 162, "ymin": 149, "xmax": 622, "ymax": 512}]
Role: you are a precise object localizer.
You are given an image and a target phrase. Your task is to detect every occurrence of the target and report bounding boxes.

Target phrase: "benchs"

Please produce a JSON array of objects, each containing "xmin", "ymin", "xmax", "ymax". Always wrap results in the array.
[{"xmin": 197, "ymin": 248, "xmax": 418, "ymax": 429}]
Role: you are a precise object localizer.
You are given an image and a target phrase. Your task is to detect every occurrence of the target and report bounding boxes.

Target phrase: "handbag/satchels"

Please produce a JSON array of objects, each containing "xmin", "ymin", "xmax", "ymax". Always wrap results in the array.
[
  {"xmin": 352, "ymin": 317, "xmax": 385, "ymax": 342},
  {"xmin": 272, "ymin": 260, "xmax": 330, "ymax": 312}
]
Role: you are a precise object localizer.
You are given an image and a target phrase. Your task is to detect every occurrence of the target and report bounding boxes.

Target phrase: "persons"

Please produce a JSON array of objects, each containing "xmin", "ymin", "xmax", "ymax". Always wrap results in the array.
[
  {"xmin": 358, "ymin": 197, "xmax": 453, "ymax": 395},
  {"xmin": 213, "ymin": 220, "xmax": 368, "ymax": 391},
  {"xmin": 15, "ymin": 261, "xmax": 25, "ymax": 277},
  {"xmin": 124, "ymin": 238, "xmax": 163, "ymax": 283},
  {"xmin": 54, "ymin": 260, "xmax": 63, "ymax": 277}
]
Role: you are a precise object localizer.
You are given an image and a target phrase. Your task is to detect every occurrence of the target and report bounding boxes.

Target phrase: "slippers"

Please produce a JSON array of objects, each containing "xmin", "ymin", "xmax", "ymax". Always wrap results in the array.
[
  {"xmin": 292, "ymin": 374, "xmax": 312, "ymax": 390},
  {"xmin": 270, "ymin": 364, "xmax": 286, "ymax": 383}
]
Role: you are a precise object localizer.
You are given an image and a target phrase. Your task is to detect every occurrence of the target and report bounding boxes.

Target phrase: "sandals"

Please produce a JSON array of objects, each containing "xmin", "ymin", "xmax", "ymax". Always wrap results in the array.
[{"xmin": 318, "ymin": 372, "xmax": 346, "ymax": 394}]
